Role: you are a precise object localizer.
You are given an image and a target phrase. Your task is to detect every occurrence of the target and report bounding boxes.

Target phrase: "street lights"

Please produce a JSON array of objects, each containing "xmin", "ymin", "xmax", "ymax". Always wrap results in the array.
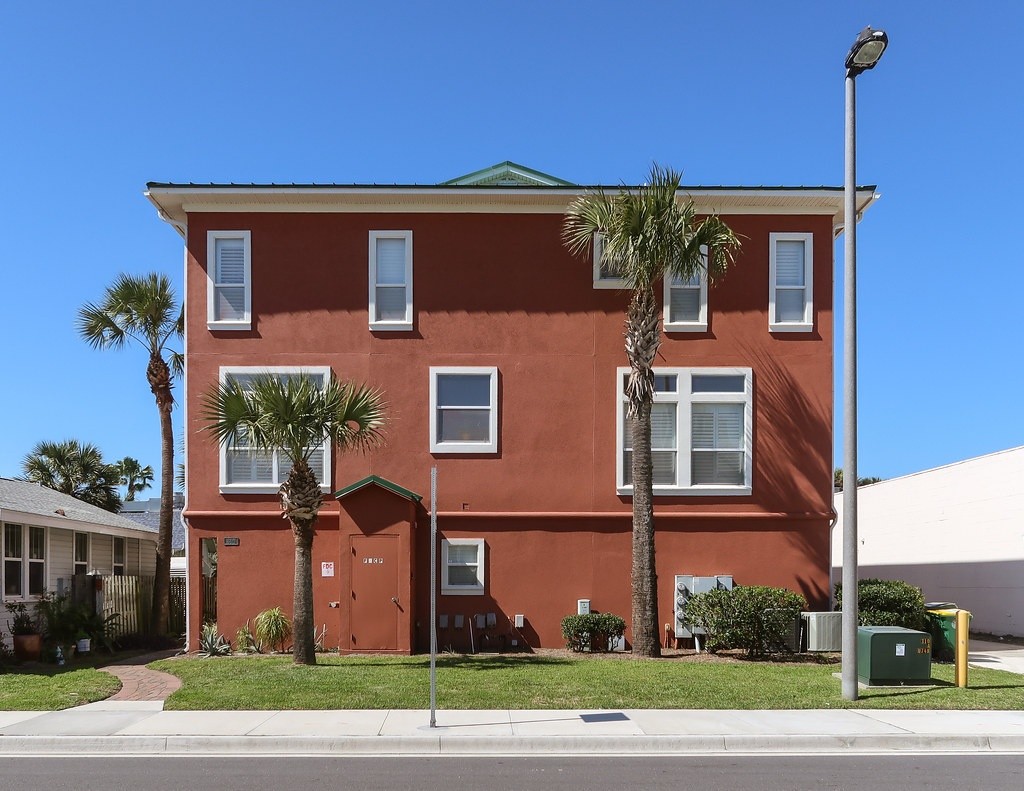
[{"xmin": 842, "ymin": 23, "xmax": 890, "ymax": 702}]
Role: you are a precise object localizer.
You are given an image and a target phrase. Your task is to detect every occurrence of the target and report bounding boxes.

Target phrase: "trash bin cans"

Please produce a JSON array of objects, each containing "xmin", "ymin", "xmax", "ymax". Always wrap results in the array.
[
  {"xmin": 926, "ymin": 609, "xmax": 973, "ymax": 664},
  {"xmin": 924, "ymin": 602, "xmax": 958, "ymax": 661}
]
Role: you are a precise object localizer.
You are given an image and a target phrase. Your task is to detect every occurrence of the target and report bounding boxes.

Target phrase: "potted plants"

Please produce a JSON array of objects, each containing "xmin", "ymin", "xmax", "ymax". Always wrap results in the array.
[{"xmin": 5, "ymin": 586, "xmax": 121, "ymax": 667}]
